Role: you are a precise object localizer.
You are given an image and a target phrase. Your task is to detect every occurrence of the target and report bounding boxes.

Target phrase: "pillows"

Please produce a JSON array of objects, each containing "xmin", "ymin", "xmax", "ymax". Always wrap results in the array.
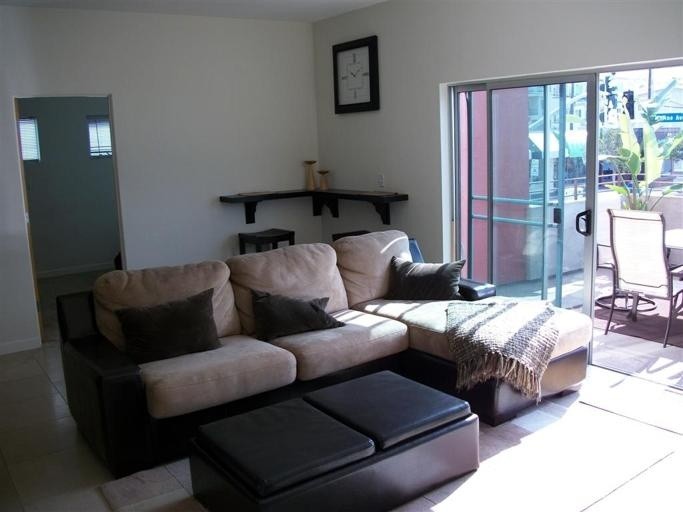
[
  {"xmin": 383, "ymin": 255, "xmax": 466, "ymax": 299},
  {"xmin": 249, "ymin": 287, "xmax": 348, "ymax": 340},
  {"xmin": 113, "ymin": 288, "xmax": 223, "ymax": 365}
]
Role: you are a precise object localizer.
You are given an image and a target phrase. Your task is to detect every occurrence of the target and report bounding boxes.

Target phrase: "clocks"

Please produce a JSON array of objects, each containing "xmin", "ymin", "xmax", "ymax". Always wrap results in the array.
[{"xmin": 333, "ymin": 34, "xmax": 379, "ymax": 113}]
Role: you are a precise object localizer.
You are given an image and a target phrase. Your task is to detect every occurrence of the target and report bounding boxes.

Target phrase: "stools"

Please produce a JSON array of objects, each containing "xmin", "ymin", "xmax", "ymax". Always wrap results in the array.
[{"xmin": 239, "ymin": 228, "xmax": 295, "ymax": 255}]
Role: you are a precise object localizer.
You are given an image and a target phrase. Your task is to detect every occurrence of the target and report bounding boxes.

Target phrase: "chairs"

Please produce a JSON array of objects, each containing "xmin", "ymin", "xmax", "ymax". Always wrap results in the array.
[{"xmin": 595, "ymin": 192, "xmax": 683, "ymax": 347}]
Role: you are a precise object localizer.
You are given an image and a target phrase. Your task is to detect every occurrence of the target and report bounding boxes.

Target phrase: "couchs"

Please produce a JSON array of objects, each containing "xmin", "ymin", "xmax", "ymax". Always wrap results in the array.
[{"xmin": 57, "ymin": 230, "xmax": 592, "ymax": 480}]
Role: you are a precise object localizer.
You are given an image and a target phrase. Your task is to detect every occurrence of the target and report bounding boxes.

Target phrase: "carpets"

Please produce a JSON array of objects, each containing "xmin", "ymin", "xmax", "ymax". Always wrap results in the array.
[{"xmin": 592, "ymin": 332, "xmax": 683, "ymax": 390}]
